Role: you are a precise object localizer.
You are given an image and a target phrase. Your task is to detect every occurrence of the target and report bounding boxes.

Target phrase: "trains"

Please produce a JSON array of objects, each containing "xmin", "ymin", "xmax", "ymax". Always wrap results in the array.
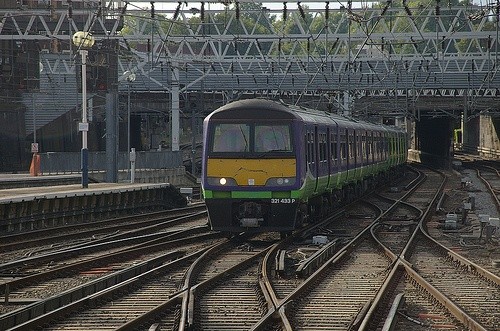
[{"xmin": 196, "ymin": 96, "xmax": 409, "ymax": 239}]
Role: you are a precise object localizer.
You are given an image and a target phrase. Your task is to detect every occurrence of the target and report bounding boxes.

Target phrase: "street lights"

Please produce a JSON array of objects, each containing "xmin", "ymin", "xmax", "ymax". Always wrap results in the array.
[
  {"xmin": 122, "ymin": 71, "xmax": 138, "ymax": 183},
  {"xmin": 74, "ymin": 31, "xmax": 96, "ymax": 188}
]
